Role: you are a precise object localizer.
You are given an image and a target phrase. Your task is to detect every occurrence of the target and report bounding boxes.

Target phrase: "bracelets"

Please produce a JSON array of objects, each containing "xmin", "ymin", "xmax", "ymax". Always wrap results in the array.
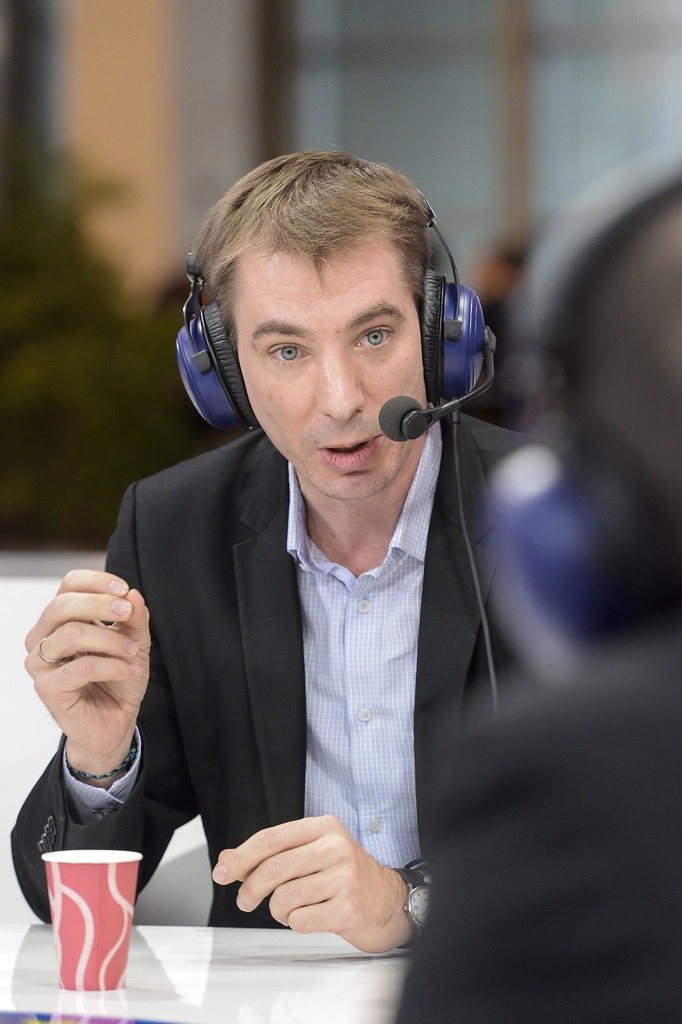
[{"xmin": 62, "ymin": 736, "xmax": 140, "ymax": 780}]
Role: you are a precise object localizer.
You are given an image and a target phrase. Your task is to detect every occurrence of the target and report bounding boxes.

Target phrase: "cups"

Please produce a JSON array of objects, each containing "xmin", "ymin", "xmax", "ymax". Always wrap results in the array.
[{"xmin": 43, "ymin": 849, "xmax": 143, "ymax": 992}]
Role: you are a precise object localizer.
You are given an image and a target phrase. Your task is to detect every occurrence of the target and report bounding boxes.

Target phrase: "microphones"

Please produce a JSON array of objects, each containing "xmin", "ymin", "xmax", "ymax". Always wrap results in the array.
[{"xmin": 378, "ymin": 326, "xmax": 497, "ymax": 443}]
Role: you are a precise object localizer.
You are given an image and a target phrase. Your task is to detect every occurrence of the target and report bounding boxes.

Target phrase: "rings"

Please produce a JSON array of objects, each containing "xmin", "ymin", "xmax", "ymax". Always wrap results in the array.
[{"xmin": 38, "ymin": 637, "xmax": 65, "ymax": 662}]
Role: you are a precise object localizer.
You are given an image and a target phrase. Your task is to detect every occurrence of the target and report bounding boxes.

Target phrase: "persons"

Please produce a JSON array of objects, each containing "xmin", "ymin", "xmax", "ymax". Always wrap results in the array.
[
  {"xmin": 10, "ymin": 152, "xmax": 576, "ymax": 955},
  {"xmin": 386, "ymin": 190, "xmax": 682, "ymax": 1020}
]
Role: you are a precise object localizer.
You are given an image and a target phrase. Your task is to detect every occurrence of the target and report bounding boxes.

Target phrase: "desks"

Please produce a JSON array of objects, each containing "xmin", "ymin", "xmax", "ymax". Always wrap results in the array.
[{"xmin": 0, "ymin": 912, "xmax": 402, "ymax": 1024}]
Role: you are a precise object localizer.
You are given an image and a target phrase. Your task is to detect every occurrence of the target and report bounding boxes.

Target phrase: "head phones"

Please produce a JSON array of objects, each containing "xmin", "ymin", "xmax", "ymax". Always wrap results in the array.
[
  {"xmin": 481, "ymin": 146, "xmax": 682, "ymax": 678},
  {"xmin": 175, "ymin": 185, "xmax": 497, "ymax": 430}
]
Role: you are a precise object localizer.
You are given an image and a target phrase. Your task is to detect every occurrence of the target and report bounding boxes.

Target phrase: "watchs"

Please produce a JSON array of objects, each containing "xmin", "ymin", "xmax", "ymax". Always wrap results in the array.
[{"xmin": 393, "ymin": 866, "xmax": 432, "ymax": 949}]
